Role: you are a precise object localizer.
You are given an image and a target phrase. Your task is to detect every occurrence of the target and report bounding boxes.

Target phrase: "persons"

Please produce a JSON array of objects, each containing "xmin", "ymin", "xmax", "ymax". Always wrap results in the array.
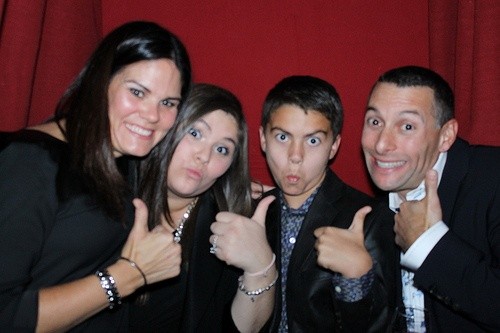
[
  {"xmin": 253, "ymin": 74, "xmax": 396, "ymax": 333},
  {"xmin": 360, "ymin": 65, "xmax": 500, "ymax": 333},
  {"xmin": 0, "ymin": 18, "xmax": 277, "ymax": 333},
  {"xmin": 131, "ymin": 82, "xmax": 281, "ymax": 333}
]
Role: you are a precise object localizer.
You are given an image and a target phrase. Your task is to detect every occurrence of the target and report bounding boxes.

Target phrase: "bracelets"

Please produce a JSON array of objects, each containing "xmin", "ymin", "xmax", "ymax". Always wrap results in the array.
[
  {"xmin": 238, "ymin": 273, "xmax": 277, "ymax": 296},
  {"xmin": 245, "ymin": 253, "xmax": 276, "ymax": 277},
  {"xmin": 95, "ymin": 268, "xmax": 122, "ymax": 309},
  {"xmin": 119, "ymin": 256, "xmax": 147, "ymax": 286}
]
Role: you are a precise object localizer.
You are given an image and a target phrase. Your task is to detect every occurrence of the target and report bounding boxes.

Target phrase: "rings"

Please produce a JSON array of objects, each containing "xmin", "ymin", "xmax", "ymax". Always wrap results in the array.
[{"xmin": 210, "ymin": 237, "xmax": 219, "ymax": 252}]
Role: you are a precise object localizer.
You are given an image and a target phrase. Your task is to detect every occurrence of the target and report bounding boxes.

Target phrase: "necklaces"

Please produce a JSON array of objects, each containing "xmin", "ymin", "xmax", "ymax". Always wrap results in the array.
[{"xmin": 173, "ymin": 196, "xmax": 198, "ymax": 243}]
